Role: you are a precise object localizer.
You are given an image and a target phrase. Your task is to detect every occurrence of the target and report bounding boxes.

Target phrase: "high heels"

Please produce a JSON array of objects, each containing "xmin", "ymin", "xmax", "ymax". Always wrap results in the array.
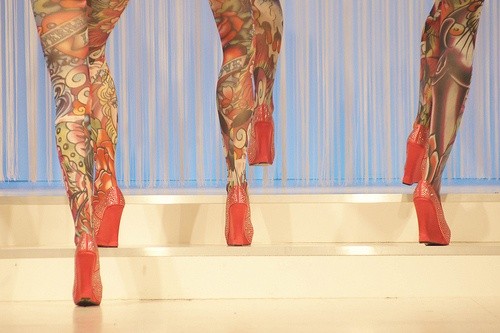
[
  {"xmin": 413, "ymin": 180, "xmax": 451, "ymax": 246},
  {"xmin": 401, "ymin": 123, "xmax": 429, "ymax": 185},
  {"xmin": 225, "ymin": 181, "xmax": 254, "ymax": 246},
  {"xmin": 247, "ymin": 105, "xmax": 275, "ymax": 166},
  {"xmin": 73, "ymin": 234, "xmax": 102, "ymax": 306},
  {"xmin": 93, "ymin": 187, "xmax": 125, "ymax": 247}
]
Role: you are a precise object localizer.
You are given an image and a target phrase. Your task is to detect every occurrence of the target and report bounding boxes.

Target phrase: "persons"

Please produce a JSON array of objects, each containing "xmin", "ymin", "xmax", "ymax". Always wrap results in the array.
[
  {"xmin": 30, "ymin": 0, "xmax": 132, "ymax": 306},
  {"xmin": 209, "ymin": 0, "xmax": 284, "ymax": 246},
  {"xmin": 401, "ymin": 0, "xmax": 484, "ymax": 247}
]
